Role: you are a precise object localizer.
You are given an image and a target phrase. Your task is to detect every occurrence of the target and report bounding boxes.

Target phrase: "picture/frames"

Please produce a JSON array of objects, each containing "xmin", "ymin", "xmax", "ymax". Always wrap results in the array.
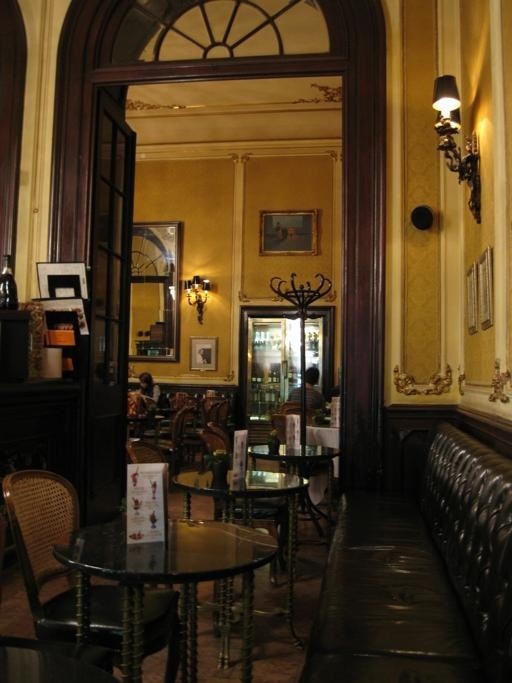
[
  {"xmin": 44, "ymin": 310, "xmax": 81, "ymax": 356},
  {"xmin": 188, "ymin": 335, "xmax": 218, "ymax": 371},
  {"xmin": 465, "ymin": 260, "xmax": 478, "ymax": 334},
  {"xmin": 258, "ymin": 208, "xmax": 319, "ymax": 256},
  {"xmin": 477, "ymin": 246, "xmax": 494, "ymax": 331}
]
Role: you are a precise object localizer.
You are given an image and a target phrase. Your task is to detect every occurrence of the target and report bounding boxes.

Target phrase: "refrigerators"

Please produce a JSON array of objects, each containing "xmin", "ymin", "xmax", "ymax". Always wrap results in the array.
[{"xmin": 246, "ymin": 317, "xmax": 324, "ymax": 444}]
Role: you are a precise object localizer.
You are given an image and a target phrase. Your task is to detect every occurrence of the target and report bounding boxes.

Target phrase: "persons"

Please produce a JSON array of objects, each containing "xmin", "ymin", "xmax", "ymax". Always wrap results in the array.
[
  {"xmin": 289, "ymin": 368, "xmax": 322, "ymax": 409},
  {"xmin": 137, "ymin": 373, "xmax": 169, "ymax": 409},
  {"xmin": 197, "ymin": 349, "xmax": 208, "ymax": 364}
]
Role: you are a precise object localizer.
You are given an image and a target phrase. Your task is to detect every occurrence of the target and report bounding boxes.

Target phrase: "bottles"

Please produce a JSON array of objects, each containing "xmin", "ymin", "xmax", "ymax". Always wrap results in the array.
[
  {"xmin": 252, "ymin": 331, "xmax": 280, "ymax": 384},
  {"xmin": 0, "ymin": 255, "xmax": 18, "ymax": 310},
  {"xmin": 252, "ymin": 386, "xmax": 280, "ymax": 402},
  {"xmin": 288, "ymin": 363, "xmax": 298, "ymax": 384},
  {"xmin": 306, "ymin": 330, "xmax": 319, "ymax": 350}
]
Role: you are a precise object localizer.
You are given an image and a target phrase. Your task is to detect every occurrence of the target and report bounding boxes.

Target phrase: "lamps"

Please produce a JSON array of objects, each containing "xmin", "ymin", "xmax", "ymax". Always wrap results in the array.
[
  {"xmin": 432, "ymin": 75, "xmax": 481, "ymax": 224},
  {"xmin": 185, "ymin": 276, "xmax": 211, "ymax": 324}
]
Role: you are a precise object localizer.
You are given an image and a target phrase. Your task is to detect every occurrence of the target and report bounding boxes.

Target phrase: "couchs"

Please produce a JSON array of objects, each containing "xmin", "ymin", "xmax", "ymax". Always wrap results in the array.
[{"xmin": 298, "ymin": 421, "xmax": 512, "ymax": 683}]
